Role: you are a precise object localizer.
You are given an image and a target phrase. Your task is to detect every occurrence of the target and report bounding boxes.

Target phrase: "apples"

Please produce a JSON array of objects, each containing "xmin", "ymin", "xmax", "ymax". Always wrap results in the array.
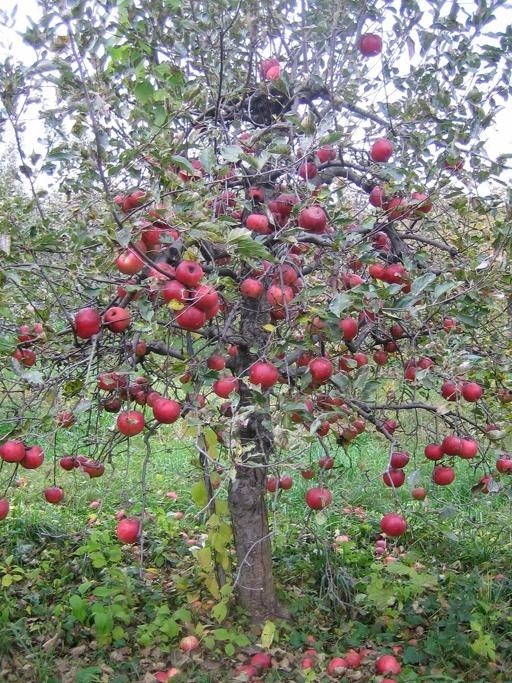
[{"xmin": 0, "ymin": 33, "xmax": 512, "ymax": 683}]
